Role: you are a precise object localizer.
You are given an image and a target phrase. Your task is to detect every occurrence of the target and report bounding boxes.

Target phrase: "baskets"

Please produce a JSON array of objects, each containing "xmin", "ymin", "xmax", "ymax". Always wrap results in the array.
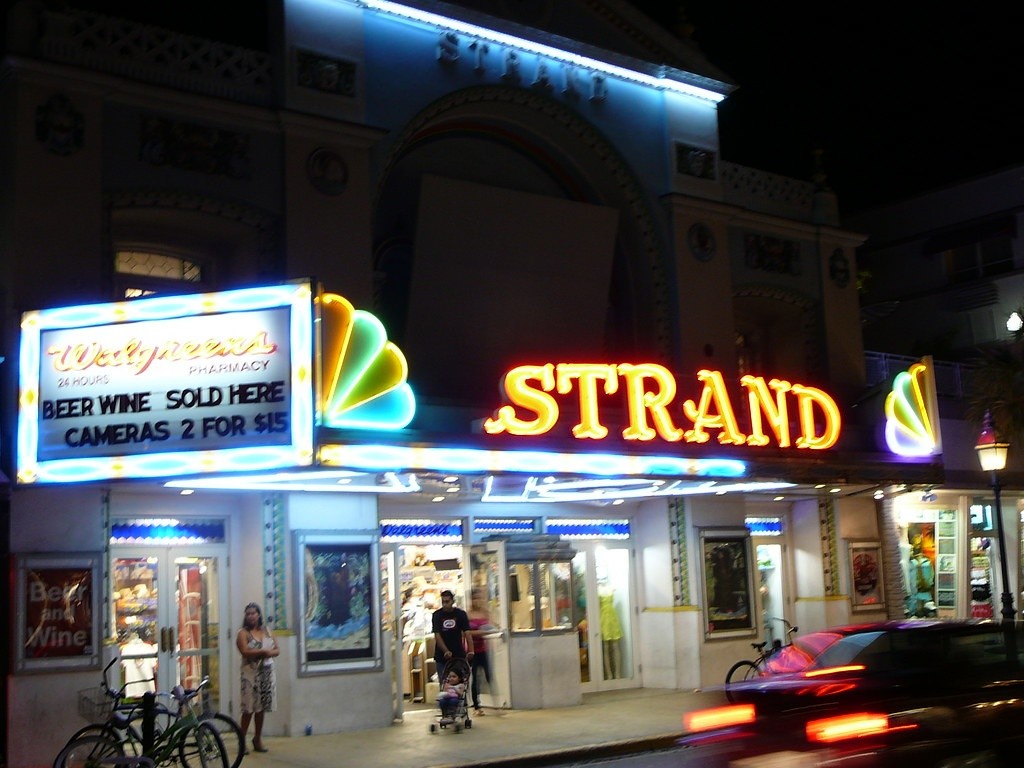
[{"xmin": 77, "ymin": 687, "xmax": 115, "ymax": 725}]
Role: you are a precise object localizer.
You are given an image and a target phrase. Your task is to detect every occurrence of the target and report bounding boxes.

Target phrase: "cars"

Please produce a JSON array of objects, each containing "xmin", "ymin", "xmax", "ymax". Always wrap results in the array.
[{"xmin": 733, "ymin": 619, "xmax": 1024, "ymax": 716}]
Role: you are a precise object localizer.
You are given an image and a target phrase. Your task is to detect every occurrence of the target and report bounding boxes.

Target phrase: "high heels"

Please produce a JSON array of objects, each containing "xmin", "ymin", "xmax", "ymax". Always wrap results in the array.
[
  {"xmin": 252, "ymin": 736, "xmax": 269, "ymax": 752},
  {"xmin": 238, "ymin": 742, "xmax": 250, "ymax": 755}
]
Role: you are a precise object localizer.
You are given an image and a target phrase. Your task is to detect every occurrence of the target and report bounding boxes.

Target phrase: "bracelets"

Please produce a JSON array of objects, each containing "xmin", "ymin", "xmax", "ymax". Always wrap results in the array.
[{"xmin": 271, "ymin": 650, "xmax": 273, "ymax": 658}]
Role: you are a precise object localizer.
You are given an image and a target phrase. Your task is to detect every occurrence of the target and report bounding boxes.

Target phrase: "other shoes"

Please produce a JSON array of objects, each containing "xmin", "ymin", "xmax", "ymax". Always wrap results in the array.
[
  {"xmin": 441, "ymin": 718, "xmax": 454, "ymax": 723},
  {"xmin": 473, "ymin": 709, "xmax": 485, "ymax": 715}
]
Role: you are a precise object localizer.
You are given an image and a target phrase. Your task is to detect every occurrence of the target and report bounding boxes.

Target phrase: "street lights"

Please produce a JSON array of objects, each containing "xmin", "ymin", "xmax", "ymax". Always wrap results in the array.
[{"xmin": 974, "ymin": 408, "xmax": 1018, "ymax": 620}]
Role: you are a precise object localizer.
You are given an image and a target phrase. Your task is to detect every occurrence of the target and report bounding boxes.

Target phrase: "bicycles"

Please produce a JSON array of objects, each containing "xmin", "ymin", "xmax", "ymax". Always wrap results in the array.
[
  {"xmin": 725, "ymin": 618, "xmax": 798, "ymax": 705},
  {"xmin": 53, "ymin": 657, "xmax": 245, "ymax": 768}
]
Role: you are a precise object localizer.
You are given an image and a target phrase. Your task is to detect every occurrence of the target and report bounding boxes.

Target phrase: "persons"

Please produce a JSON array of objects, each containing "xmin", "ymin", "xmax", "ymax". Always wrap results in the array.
[
  {"xmin": 462, "ymin": 606, "xmax": 505, "ymax": 716},
  {"xmin": 237, "ymin": 603, "xmax": 280, "ymax": 754},
  {"xmin": 597, "ymin": 573, "xmax": 622, "ymax": 680},
  {"xmin": 431, "ymin": 589, "xmax": 474, "ymax": 692},
  {"xmin": 438, "ymin": 669, "xmax": 465, "ymax": 724},
  {"xmin": 706, "ymin": 544, "xmax": 749, "ymax": 616}
]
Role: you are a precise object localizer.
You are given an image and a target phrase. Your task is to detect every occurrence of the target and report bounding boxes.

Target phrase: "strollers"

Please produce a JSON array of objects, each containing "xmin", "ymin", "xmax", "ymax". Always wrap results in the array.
[{"xmin": 430, "ymin": 655, "xmax": 472, "ymax": 732}]
